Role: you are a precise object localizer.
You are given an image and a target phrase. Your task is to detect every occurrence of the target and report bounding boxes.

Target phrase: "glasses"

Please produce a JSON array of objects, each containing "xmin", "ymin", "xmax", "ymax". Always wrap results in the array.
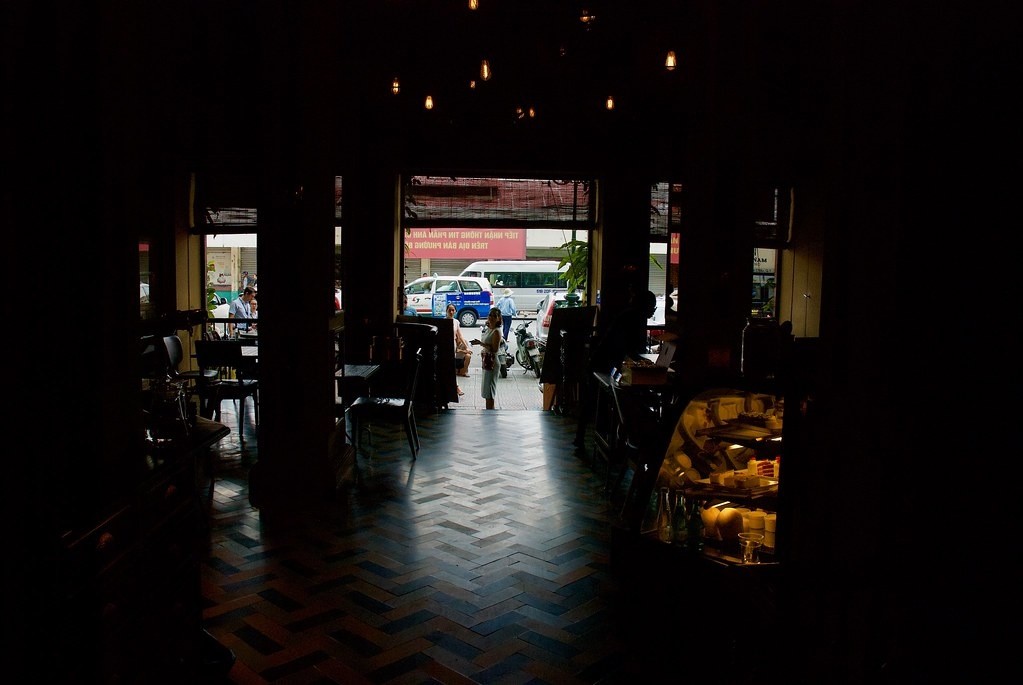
[
  {"xmin": 488, "ymin": 313, "xmax": 501, "ymax": 318},
  {"xmin": 406, "ymin": 301, "xmax": 407, "ymax": 303}
]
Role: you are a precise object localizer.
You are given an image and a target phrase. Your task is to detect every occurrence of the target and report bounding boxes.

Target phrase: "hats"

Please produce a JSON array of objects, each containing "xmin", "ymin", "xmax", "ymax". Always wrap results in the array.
[{"xmin": 503, "ymin": 288, "xmax": 515, "ymax": 297}]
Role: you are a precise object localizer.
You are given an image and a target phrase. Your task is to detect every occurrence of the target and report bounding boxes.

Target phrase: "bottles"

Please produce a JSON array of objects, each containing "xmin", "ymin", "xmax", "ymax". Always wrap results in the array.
[
  {"xmin": 656, "ymin": 487, "xmax": 671, "ymax": 543},
  {"xmin": 750, "ymin": 457, "xmax": 758, "ymax": 474},
  {"xmin": 687, "ymin": 495, "xmax": 705, "ymax": 554},
  {"xmin": 596, "ymin": 289, "xmax": 601, "ymax": 311},
  {"xmin": 670, "ymin": 490, "xmax": 688, "ymax": 554},
  {"xmin": 773, "ymin": 456, "xmax": 780, "ymax": 477}
]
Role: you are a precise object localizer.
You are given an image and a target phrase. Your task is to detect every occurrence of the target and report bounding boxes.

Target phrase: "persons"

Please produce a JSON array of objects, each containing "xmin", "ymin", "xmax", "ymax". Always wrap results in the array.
[
  {"xmin": 444, "ymin": 303, "xmax": 473, "ymax": 395},
  {"xmin": 496, "ymin": 288, "xmax": 517, "ymax": 342},
  {"xmin": 241, "ymin": 271, "xmax": 257, "ymax": 293},
  {"xmin": 471, "ymin": 306, "xmax": 503, "ymax": 410},
  {"xmin": 423, "ymin": 283, "xmax": 430, "ymax": 294},
  {"xmin": 403, "ymin": 295, "xmax": 418, "ymax": 316},
  {"xmin": 574, "ymin": 290, "xmax": 656, "ymax": 449},
  {"xmin": 494, "ymin": 276, "xmax": 502, "ymax": 285},
  {"xmin": 228, "ymin": 286, "xmax": 258, "ymax": 338},
  {"xmin": 423, "ymin": 273, "xmax": 427, "ymax": 277},
  {"xmin": 335, "ymin": 280, "xmax": 342, "ymax": 309}
]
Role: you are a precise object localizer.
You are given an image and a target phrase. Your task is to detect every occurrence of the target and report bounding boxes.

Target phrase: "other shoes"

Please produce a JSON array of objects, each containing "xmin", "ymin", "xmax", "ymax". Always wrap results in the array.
[{"xmin": 457, "ymin": 386, "xmax": 465, "ymax": 396}]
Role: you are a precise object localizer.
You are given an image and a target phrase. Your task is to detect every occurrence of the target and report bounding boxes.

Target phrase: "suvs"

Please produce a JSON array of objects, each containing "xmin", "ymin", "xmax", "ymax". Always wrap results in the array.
[{"xmin": 535, "ymin": 292, "xmax": 583, "ymax": 352}]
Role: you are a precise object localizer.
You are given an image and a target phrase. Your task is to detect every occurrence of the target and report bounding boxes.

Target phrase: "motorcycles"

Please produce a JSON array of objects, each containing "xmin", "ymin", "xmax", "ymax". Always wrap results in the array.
[{"xmin": 481, "ymin": 315, "xmax": 540, "ymax": 378}]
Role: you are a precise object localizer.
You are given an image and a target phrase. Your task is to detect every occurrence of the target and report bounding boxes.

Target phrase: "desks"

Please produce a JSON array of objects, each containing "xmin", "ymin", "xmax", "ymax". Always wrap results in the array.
[
  {"xmin": 592, "ymin": 370, "xmax": 678, "ymax": 492},
  {"xmin": 239, "ymin": 346, "xmax": 258, "ymax": 378},
  {"xmin": 341, "ymin": 363, "xmax": 382, "ymax": 428}
]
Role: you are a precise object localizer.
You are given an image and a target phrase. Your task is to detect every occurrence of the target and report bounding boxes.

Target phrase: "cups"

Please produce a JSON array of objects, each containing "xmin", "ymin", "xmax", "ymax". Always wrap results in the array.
[
  {"xmin": 738, "ymin": 532, "xmax": 764, "ymax": 564},
  {"xmin": 764, "ymin": 514, "xmax": 777, "ymax": 532},
  {"xmin": 743, "ymin": 510, "xmax": 767, "ymax": 529}
]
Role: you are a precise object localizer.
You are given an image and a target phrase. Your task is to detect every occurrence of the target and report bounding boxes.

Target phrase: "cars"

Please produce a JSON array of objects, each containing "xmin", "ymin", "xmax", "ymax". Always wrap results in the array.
[{"xmin": 207, "ymin": 292, "xmax": 230, "ymax": 338}]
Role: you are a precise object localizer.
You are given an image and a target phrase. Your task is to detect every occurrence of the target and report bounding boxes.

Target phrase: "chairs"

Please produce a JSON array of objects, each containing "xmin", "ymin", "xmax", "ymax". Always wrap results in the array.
[{"xmin": 131, "ymin": 330, "xmax": 422, "ymax": 460}]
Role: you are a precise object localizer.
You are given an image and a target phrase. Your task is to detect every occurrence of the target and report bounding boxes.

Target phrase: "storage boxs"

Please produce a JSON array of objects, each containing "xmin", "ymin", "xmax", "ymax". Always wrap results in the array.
[{"xmin": 622, "ymin": 363, "xmax": 668, "ymax": 386}]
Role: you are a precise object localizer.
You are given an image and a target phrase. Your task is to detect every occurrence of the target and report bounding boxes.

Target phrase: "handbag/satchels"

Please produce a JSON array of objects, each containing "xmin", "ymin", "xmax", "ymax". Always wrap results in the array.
[{"xmin": 480, "ymin": 352, "xmax": 496, "ymax": 371}]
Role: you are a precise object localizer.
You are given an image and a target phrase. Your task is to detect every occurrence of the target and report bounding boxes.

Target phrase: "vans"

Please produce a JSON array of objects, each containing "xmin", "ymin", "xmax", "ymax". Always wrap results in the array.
[
  {"xmin": 459, "ymin": 261, "xmax": 583, "ymax": 314},
  {"xmin": 404, "ymin": 276, "xmax": 494, "ymax": 327}
]
